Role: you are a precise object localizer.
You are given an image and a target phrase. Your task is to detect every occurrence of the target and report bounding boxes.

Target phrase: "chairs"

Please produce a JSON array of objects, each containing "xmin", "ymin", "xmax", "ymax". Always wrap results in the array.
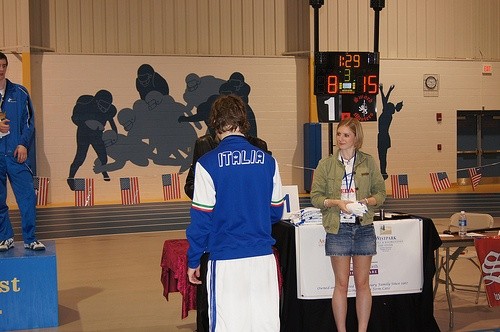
[{"xmin": 435, "ymin": 212, "xmax": 494, "ymax": 304}]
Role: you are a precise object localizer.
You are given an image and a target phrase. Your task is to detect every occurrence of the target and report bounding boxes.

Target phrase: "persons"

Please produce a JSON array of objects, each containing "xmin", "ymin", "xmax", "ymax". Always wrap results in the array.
[
  {"xmin": 310, "ymin": 117, "xmax": 386, "ymax": 332},
  {"xmin": 0, "ymin": 51, "xmax": 46, "ymax": 251},
  {"xmin": 184, "ymin": 133, "xmax": 271, "ymax": 201},
  {"xmin": 187, "ymin": 96, "xmax": 282, "ymax": 332}
]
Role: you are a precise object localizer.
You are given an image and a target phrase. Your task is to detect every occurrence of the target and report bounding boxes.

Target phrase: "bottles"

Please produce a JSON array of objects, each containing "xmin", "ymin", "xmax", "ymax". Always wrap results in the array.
[{"xmin": 458, "ymin": 211, "xmax": 467, "ymax": 236}]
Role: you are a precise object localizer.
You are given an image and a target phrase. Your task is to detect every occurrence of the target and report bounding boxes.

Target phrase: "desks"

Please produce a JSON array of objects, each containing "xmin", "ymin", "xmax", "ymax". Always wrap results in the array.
[
  {"xmin": 271, "ymin": 210, "xmax": 442, "ymax": 332},
  {"xmin": 160, "ymin": 239, "xmax": 283, "ymax": 319},
  {"xmin": 436, "ymin": 232, "xmax": 500, "ymax": 330}
]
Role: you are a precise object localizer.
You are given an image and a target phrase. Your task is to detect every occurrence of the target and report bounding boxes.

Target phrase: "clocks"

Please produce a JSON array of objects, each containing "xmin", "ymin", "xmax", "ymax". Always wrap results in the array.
[{"xmin": 426, "ymin": 76, "xmax": 437, "ymax": 89}]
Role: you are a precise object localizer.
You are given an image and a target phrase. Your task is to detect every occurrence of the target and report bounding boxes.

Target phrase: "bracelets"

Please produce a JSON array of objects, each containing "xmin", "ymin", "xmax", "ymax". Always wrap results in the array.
[
  {"xmin": 324, "ymin": 199, "xmax": 328, "ymax": 207},
  {"xmin": 365, "ymin": 198, "xmax": 368, "ymax": 206}
]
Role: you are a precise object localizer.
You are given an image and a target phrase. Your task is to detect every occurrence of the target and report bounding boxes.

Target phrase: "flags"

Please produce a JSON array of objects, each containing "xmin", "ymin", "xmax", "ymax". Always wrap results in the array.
[
  {"xmin": 391, "ymin": 174, "xmax": 409, "ymax": 199},
  {"xmin": 74, "ymin": 178, "xmax": 94, "ymax": 206},
  {"xmin": 120, "ymin": 177, "xmax": 139, "ymax": 206},
  {"xmin": 430, "ymin": 172, "xmax": 450, "ymax": 192},
  {"xmin": 469, "ymin": 166, "xmax": 484, "ymax": 191},
  {"xmin": 162, "ymin": 172, "xmax": 181, "ymax": 201},
  {"xmin": 33, "ymin": 176, "xmax": 48, "ymax": 206}
]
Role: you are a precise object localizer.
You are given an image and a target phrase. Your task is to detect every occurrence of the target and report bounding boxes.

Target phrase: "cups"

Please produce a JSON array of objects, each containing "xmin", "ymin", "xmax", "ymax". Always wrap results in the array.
[{"xmin": 380, "ymin": 209, "xmax": 384, "ymax": 220}]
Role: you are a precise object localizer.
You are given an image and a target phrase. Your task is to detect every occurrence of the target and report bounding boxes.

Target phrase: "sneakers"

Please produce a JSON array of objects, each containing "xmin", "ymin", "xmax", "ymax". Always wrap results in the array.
[
  {"xmin": 24, "ymin": 240, "xmax": 46, "ymax": 251},
  {"xmin": 0, "ymin": 236, "xmax": 14, "ymax": 251}
]
